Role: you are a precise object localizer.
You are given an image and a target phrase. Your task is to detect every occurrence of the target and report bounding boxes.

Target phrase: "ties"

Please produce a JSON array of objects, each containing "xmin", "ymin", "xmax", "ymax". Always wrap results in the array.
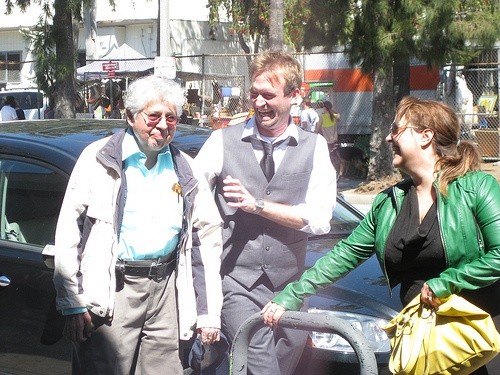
[{"xmin": 260, "ymin": 140, "xmax": 285, "ymax": 183}]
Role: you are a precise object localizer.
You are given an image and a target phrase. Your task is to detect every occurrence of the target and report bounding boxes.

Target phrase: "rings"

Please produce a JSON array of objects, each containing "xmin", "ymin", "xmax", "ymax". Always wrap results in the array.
[
  {"xmin": 207, "ymin": 337, "xmax": 213, "ymax": 340},
  {"xmin": 427, "ymin": 296, "xmax": 433, "ymax": 301},
  {"xmin": 270, "ymin": 308, "xmax": 274, "ymax": 313}
]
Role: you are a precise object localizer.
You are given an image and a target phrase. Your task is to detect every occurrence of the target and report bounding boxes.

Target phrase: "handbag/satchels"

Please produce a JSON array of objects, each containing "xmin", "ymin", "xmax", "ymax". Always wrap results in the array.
[{"xmin": 382, "ymin": 292, "xmax": 500, "ymax": 375}]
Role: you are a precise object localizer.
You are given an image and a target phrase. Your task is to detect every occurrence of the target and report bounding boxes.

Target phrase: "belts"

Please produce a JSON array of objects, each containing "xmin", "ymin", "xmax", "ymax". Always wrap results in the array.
[{"xmin": 124, "ymin": 257, "xmax": 177, "ymax": 277}]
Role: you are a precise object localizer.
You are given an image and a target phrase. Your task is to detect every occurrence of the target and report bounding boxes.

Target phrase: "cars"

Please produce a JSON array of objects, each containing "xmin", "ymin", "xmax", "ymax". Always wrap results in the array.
[{"xmin": 0, "ymin": 117, "xmax": 402, "ymax": 375}]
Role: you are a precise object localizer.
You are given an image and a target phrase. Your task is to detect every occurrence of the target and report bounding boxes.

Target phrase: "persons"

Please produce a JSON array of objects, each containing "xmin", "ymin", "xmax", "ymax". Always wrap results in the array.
[
  {"xmin": 193, "ymin": 49, "xmax": 338, "ymax": 375},
  {"xmin": 289, "ymin": 86, "xmax": 342, "ymax": 182},
  {"xmin": 52, "ymin": 74, "xmax": 224, "ymax": 375},
  {"xmin": 259, "ymin": 95, "xmax": 500, "ymax": 375},
  {"xmin": 0, "ymin": 94, "xmax": 125, "ymax": 122},
  {"xmin": 228, "ymin": 98, "xmax": 250, "ymax": 116}
]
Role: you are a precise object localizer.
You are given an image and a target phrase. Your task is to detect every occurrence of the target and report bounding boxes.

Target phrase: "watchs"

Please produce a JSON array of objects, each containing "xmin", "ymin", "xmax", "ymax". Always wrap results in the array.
[{"xmin": 253, "ymin": 197, "xmax": 264, "ymax": 216}]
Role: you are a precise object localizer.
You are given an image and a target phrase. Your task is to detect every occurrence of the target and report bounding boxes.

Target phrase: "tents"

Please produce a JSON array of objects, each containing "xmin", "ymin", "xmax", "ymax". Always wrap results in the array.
[{"xmin": 74, "ymin": 43, "xmax": 246, "ymax": 113}]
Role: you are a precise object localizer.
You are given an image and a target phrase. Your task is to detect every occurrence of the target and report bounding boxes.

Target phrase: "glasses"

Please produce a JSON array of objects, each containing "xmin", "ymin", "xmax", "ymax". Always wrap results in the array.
[
  {"xmin": 390, "ymin": 122, "xmax": 420, "ymax": 135},
  {"xmin": 142, "ymin": 110, "xmax": 182, "ymax": 125}
]
